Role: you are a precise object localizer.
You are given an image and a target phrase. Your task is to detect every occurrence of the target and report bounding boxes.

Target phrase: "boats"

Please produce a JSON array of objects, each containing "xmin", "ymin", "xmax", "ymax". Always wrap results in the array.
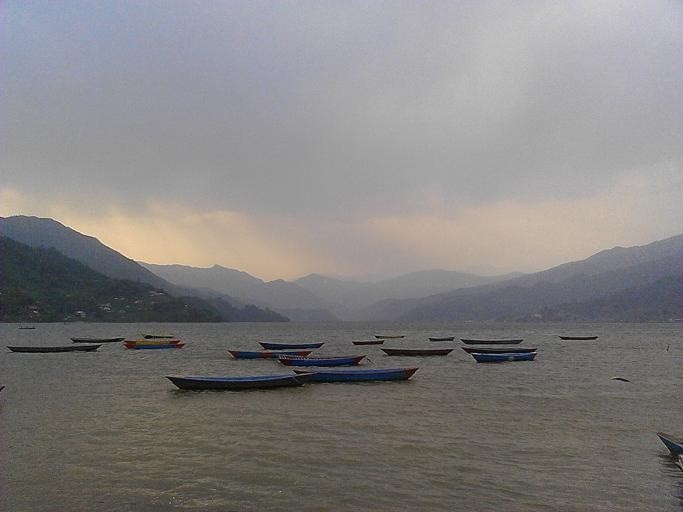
[
  {"xmin": 471, "ymin": 352, "xmax": 537, "ymax": 363},
  {"xmin": 558, "ymin": 335, "xmax": 597, "ymax": 340},
  {"xmin": 124, "ymin": 340, "xmax": 186, "ymax": 349},
  {"xmin": 165, "ymin": 371, "xmax": 321, "ymax": 391},
  {"xmin": 375, "ymin": 335, "xmax": 407, "ymax": 339},
  {"xmin": 654, "ymin": 430, "xmax": 683, "ymax": 458},
  {"xmin": 428, "ymin": 336, "xmax": 455, "ymax": 341},
  {"xmin": 227, "ymin": 349, "xmax": 312, "ymax": 360},
  {"xmin": 259, "ymin": 340, "xmax": 325, "ymax": 350},
  {"xmin": 461, "ymin": 346, "xmax": 537, "ymax": 354},
  {"xmin": 141, "ymin": 333, "xmax": 174, "ymax": 339},
  {"xmin": 277, "ymin": 354, "xmax": 367, "ymax": 367},
  {"xmin": 70, "ymin": 336, "xmax": 126, "ymax": 343},
  {"xmin": 351, "ymin": 340, "xmax": 385, "ymax": 345},
  {"xmin": 381, "ymin": 347, "xmax": 453, "ymax": 357},
  {"xmin": 18, "ymin": 325, "xmax": 36, "ymax": 330},
  {"xmin": 460, "ymin": 338, "xmax": 523, "ymax": 345},
  {"xmin": 7, "ymin": 344, "xmax": 102, "ymax": 353},
  {"xmin": 293, "ymin": 367, "xmax": 419, "ymax": 383}
]
